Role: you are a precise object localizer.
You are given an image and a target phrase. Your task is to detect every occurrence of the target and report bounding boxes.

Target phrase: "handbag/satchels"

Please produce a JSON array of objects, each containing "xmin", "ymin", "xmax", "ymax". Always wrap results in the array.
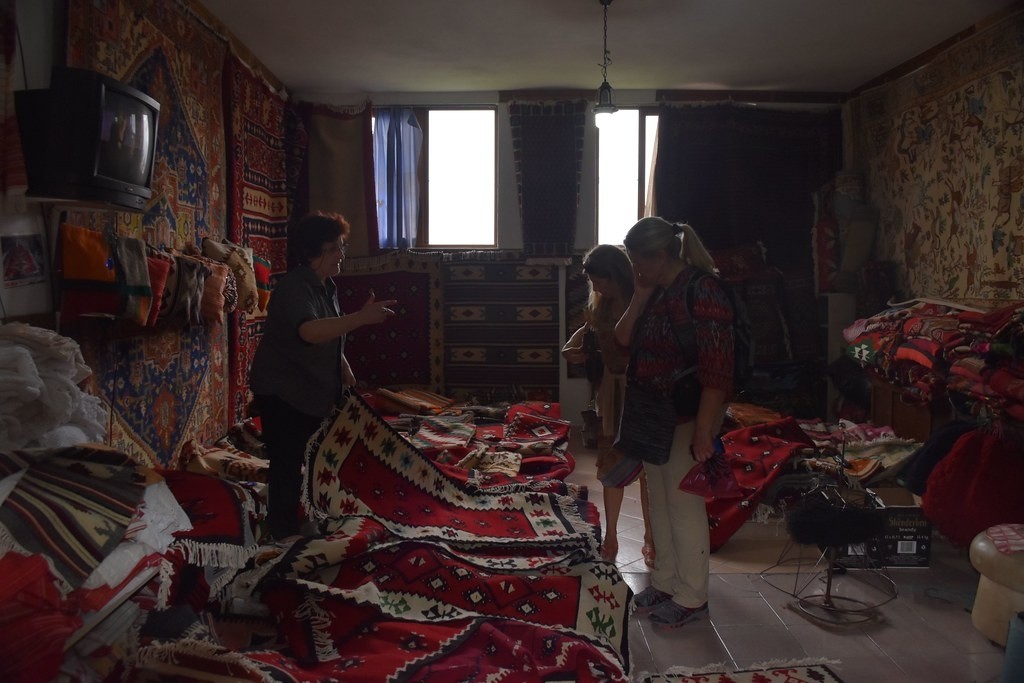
[
  {"xmin": 612, "ymin": 382, "xmax": 676, "ymax": 466},
  {"xmin": 581, "ymin": 328, "xmax": 605, "ymax": 382}
]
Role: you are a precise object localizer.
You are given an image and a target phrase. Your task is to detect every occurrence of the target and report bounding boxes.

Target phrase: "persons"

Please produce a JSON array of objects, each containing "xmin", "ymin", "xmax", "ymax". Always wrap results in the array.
[
  {"xmin": 561, "ymin": 244, "xmax": 656, "ymax": 568},
  {"xmin": 249, "ymin": 210, "xmax": 400, "ymax": 542},
  {"xmin": 614, "ymin": 213, "xmax": 735, "ymax": 628}
]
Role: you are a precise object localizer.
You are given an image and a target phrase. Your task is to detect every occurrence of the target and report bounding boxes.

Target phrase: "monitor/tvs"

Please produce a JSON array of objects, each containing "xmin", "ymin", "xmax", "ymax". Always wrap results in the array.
[{"xmin": 16, "ymin": 67, "xmax": 160, "ymax": 210}]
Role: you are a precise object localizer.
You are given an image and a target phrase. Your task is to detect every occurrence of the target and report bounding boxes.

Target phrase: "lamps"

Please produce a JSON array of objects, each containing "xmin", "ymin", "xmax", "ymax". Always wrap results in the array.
[{"xmin": 588, "ymin": 0, "xmax": 620, "ymax": 126}]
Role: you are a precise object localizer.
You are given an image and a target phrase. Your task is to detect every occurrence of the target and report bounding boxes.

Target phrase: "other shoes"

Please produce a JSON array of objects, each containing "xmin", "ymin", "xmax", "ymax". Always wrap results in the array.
[
  {"xmin": 600, "ymin": 543, "xmax": 619, "ymax": 563},
  {"xmin": 642, "ymin": 545, "xmax": 656, "ymax": 567}
]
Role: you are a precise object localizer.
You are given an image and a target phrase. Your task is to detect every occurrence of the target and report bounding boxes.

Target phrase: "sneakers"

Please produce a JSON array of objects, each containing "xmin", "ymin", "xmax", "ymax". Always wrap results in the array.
[
  {"xmin": 633, "ymin": 585, "xmax": 674, "ymax": 611},
  {"xmin": 648, "ymin": 600, "xmax": 709, "ymax": 628}
]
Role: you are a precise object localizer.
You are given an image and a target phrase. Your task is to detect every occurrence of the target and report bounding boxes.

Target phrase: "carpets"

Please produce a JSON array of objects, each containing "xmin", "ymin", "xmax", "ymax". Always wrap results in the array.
[{"xmin": 58, "ymin": 0, "xmax": 850, "ymax": 683}]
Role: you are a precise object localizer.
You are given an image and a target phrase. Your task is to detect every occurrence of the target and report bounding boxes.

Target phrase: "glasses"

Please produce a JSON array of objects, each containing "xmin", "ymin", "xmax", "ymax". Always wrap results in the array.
[{"xmin": 320, "ymin": 243, "xmax": 349, "ymax": 257}]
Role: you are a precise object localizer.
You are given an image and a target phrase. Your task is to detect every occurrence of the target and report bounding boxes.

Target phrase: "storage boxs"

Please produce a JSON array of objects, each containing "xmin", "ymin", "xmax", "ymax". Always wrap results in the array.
[{"xmin": 864, "ymin": 488, "xmax": 933, "ymax": 569}]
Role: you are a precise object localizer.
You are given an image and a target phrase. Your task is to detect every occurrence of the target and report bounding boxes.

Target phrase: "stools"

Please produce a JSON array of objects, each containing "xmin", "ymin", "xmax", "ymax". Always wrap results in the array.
[{"xmin": 969, "ymin": 523, "xmax": 1024, "ymax": 648}]
